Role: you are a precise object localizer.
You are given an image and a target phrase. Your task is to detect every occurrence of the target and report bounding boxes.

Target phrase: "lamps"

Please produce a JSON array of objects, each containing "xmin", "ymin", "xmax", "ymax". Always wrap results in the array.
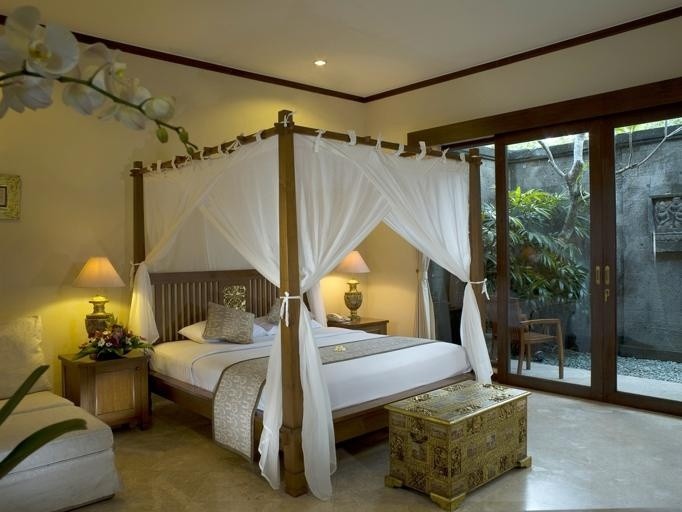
[
  {"xmin": 335, "ymin": 249, "xmax": 372, "ymax": 320},
  {"xmin": 72, "ymin": 254, "xmax": 128, "ymax": 336}
]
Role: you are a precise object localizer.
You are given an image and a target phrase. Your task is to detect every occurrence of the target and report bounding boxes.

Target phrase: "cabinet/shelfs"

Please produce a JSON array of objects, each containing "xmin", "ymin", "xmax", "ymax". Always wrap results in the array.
[{"xmin": 384, "ymin": 378, "xmax": 533, "ymax": 512}]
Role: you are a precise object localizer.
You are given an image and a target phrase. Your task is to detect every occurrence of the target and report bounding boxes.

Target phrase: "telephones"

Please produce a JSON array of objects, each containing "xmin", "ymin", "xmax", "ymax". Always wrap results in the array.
[{"xmin": 327, "ymin": 313, "xmax": 351, "ymax": 323}]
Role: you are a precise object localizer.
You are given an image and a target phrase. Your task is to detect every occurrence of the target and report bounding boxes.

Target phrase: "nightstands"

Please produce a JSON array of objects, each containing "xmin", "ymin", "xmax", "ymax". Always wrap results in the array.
[
  {"xmin": 328, "ymin": 317, "xmax": 390, "ymax": 335},
  {"xmin": 58, "ymin": 347, "xmax": 154, "ymax": 435}
]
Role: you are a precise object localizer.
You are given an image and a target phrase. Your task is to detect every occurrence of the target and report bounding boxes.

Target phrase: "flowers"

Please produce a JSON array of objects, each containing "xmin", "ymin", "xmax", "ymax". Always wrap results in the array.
[
  {"xmin": 70, "ymin": 325, "xmax": 152, "ymax": 361},
  {"xmin": 0, "ymin": 0, "xmax": 204, "ymax": 479}
]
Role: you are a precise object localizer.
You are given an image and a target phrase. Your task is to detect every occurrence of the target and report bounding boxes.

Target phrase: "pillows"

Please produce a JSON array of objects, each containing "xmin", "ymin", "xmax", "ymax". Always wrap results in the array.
[
  {"xmin": 202, "ymin": 299, "xmax": 257, "ymax": 346},
  {"xmin": 0, "ymin": 314, "xmax": 51, "ymax": 395},
  {"xmin": 177, "ymin": 316, "xmax": 267, "ymax": 343}
]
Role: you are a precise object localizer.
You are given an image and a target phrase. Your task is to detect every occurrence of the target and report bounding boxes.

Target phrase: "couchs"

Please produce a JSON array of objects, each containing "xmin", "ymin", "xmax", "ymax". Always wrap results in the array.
[{"xmin": 0, "ymin": 388, "xmax": 124, "ymax": 512}]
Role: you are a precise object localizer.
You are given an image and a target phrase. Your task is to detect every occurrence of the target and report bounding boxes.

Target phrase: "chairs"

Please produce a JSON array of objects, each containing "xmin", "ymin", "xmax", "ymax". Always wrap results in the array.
[{"xmin": 487, "ymin": 294, "xmax": 565, "ymax": 380}]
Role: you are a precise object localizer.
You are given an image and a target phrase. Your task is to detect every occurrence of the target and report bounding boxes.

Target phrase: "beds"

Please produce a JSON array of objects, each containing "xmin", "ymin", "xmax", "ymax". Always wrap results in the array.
[{"xmin": 128, "ymin": 106, "xmax": 490, "ymax": 498}]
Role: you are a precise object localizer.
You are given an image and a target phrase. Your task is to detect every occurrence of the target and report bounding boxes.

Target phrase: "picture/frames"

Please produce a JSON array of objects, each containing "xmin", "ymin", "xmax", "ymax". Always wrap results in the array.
[{"xmin": 0, "ymin": 174, "xmax": 21, "ymax": 223}]
